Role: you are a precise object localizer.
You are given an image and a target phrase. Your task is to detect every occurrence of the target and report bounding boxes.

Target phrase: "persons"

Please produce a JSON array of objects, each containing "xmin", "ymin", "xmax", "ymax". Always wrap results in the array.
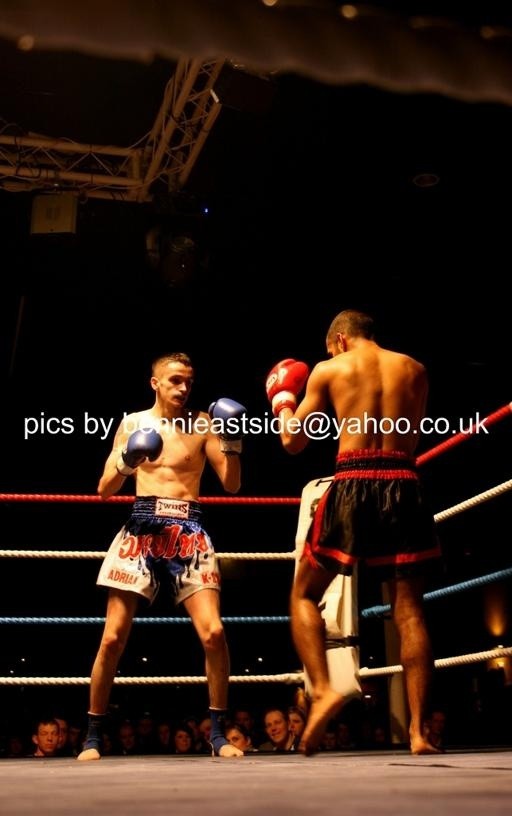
[
  {"xmin": 77, "ymin": 352, "xmax": 247, "ymax": 758},
  {"xmin": 266, "ymin": 309, "xmax": 441, "ymax": 754},
  {"xmin": 0, "ymin": 705, "xmax": 447, "ymax": 758}
]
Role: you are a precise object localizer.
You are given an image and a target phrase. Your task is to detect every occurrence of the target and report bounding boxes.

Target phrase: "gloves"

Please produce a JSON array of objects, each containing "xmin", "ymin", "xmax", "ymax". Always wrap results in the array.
[
  {"xmin": 112, "ymin": 427, "xmax": 162, "ymax": 475},
  {"xmin": 265, "ymin": 358, "xmax": 309, "ymax": 415},
  {"xmin": 208, "ymin": 398, "xmax": 249, "ymax": 456}
]
